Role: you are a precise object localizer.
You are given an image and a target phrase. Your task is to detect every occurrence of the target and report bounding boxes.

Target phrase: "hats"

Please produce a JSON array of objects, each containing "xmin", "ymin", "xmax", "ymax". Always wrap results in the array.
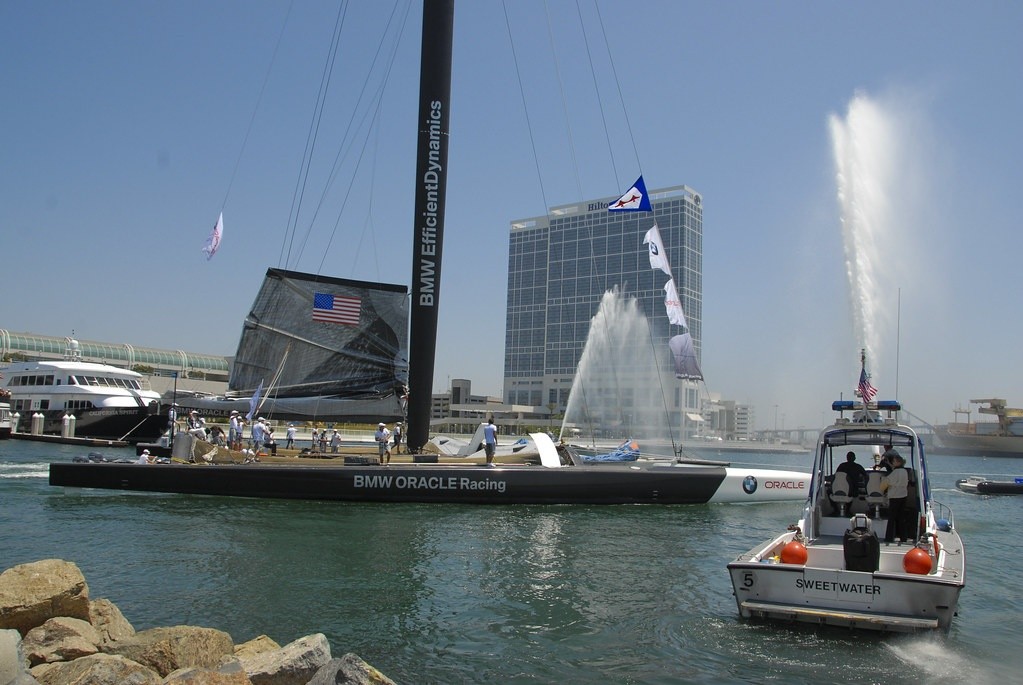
[
  {"xmin": 258, "ymin": 417, "xmax": 266, "ymax": 421},
  {"xmin": 378, "ymin": 423, "xmax": 386, "ymax": 428},
  {"xmin": 396, "ymin": 421, "xmax": 402, "ymax": 424},
  {"xmin": 231, "ymin": 410, "xmax": 238, "ymax": 414},
  {"xmin": 143, "ymin": 449, "xmax": 150, "ymax": 452},
  {"xmin": 323, "ymin": 429, "xmax": 327, "ymax": 431},
  {"xmin": 290, "ymin": 423, "xmax": 293, "ymax": 426},
  {"xmin": 172, "ymin": 402, "xmax": 179, "ymax": 406},
  {"xmin": 237, "ymin": 416, "xmax": 242, "ymax": 419},
  {"xmin": 333, "ymin": 429, "xmax": 337, "ymax": 431},
  {"xmin": 266, "ymin": 422, "xmax": 271, "ymax": 424}
]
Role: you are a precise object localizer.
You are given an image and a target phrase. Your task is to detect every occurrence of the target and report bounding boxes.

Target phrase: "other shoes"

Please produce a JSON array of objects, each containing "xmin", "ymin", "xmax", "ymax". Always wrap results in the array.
[{"xmin": 486, "ymin": 463, "xmax": 496, "ymax": 469}]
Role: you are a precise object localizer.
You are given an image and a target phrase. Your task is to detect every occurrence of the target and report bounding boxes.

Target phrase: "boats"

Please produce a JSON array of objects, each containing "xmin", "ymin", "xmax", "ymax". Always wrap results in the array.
[
  {"xmin": 954, "ymin": 474, "xmax": 1023, "ymax": 495},
  {"xmin": 0, "ymin": 402, "xmax": 13, "ymax": 440},
  {"xmin": 0, "ymin": 339, "xmax": 178, "ymax": 445},
  {"xmin": 933, "ymin": 399, "xmax": 1023, "ymax": 458},
  {"xmin": 653, "ymin": 458, "xmax": 813, "ymax": 505},
  {"xmin": 726, "ymin": 285, "xmax": 966, "ymax": 642}
]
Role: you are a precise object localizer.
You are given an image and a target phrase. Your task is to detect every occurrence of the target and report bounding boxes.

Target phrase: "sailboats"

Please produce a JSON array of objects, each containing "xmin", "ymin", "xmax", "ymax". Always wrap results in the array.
[{"xmin": 49, "ymin": 0, "xmax": 729, "ymax": 508}]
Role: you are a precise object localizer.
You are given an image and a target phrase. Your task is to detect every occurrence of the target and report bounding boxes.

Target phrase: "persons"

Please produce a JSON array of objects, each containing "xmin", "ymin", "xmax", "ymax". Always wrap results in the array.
[
  {"xmin": 311, "ymin": 429, "xmax": 341, "ymax": 453},
  {"xmin": 375, "ymin": 423, "xmax": 391, "ymax": 466},
  {"xmin": 169, "ymin": 403, "xmax": 179, "ymax": 439},
  {"xmin": 285, "ymin": 424, "xmax": 297, "ymax": 450},
  {"xmin": 253, "ymin": 417, "xmax": 278, "ymax": 462},
  {"xmin": 229, "ymin": 410, "xmax": 244, "ymax": 452},
  {"xmin": 386, "ymin": 422, "xmax": 402, "ymax": 454},
  {"xmin": 836, "ymin": 451, "xmax": 868, "ymax": 484},
  {"xmin": 879, "ymin": 455, "xmax": 908, "ymax": 543},
  {"xmin": 484, "ymin": 418, "xmax": 497, "ymax": 468},
  {"xmin": 139, "ymin": 449, "xmax": 152, "ymax": 465},
  {"xmin": 186, "ymin": 410, "xmax": 226, "ymax": 446},
  {"xmin": 875, "ymin": 445, "xmax": 899, "ymax": 472}
]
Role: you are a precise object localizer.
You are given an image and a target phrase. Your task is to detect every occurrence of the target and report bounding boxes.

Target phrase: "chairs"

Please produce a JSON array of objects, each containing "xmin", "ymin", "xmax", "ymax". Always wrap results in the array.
[
  {"xmin": 830, "ymin": 472, "xmax": 853, "ymax": 516},
  {"xmin": 865, "ymin": 471, "xmax": 885, "ymax": 521}
]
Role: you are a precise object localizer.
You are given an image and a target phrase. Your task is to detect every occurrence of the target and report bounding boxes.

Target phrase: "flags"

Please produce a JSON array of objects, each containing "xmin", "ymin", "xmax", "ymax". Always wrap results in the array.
[
  {"xmin": 663, "ymin": 279, "xmax": 687, "ymax": 328},
  {"xmin": 201, "ymin": 211, "xmax": 223, "ymax": 261},
  {"xmin": 669, "ymin": 332, "xmax": 704, "ymax": 381},
  {"xmin": 643, "ymin": 225, "xmax": 671, "ymax": 275},
  {"xmin": 858, "ymin": 368, "xmax": 878, "ymax": 405},
  {"xmin": 608, "ymin": 174, "xmax": 652, "ymax": 212},
  {"xmin": 312, "ymin": 292, "xmax": 362, "ymax": 325}
]
